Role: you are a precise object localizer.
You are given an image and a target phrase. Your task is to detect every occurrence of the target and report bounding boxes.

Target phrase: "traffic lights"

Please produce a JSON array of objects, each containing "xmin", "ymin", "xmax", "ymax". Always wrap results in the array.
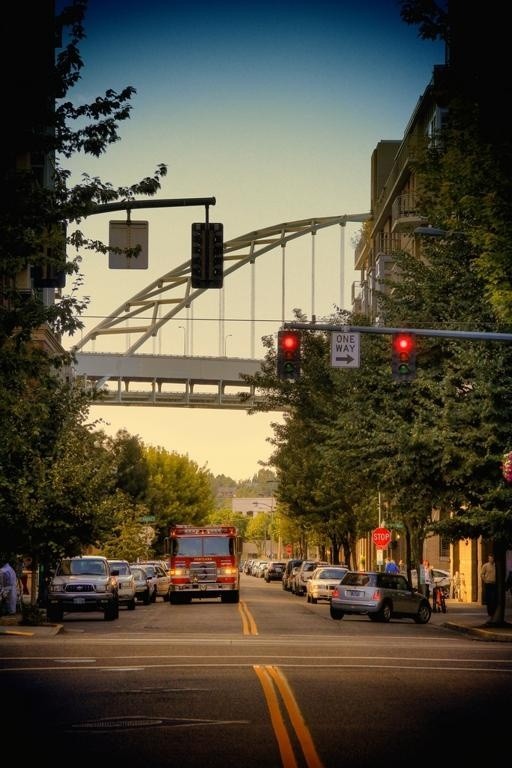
[
  {"xmin": 392, "ymin": 335, "xmax": 415, "ymax": 375},
  {"xmin": 278, "ymin": 330, "xmax": 300, "ymax": 377}
]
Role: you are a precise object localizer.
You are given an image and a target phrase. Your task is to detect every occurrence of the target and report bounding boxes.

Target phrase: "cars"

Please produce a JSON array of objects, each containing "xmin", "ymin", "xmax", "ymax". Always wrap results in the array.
[
  {"xmin": 107, "ymin": 558, "xmax": 169, "ymax": 610},
  {"xmin": 329, "ymin": 569, "xmax": 432, "ymax": 624},
  {"xmin": 240, "ymin": 559, "xmax": 352, "ymax": 603}
]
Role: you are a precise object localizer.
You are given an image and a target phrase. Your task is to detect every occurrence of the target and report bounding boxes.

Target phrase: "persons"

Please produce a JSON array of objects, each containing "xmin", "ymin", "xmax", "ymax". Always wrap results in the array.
[
  {"xmin": 423, "ymin": 560, "xmax": 435, "ymax": 599},
  {"xmin": 383, "ymin": 558, "xmax": 404, "ymax": 575},
  {"xmin": 480, "ymin": 553, "xmax": 498, "ymax": 620}
]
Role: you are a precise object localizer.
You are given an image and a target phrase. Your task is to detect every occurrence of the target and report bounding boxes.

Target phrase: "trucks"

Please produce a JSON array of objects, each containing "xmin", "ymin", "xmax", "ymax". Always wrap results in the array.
[{"xmin": 167, "ymin": 524, "xmax": 240, "ymax": 605}]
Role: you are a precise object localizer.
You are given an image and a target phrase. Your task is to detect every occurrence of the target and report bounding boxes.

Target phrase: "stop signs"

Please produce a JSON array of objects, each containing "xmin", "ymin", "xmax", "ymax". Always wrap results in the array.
[{"xmin": 371, "ymin": 527, "xmax": 391, "ymax": 546}]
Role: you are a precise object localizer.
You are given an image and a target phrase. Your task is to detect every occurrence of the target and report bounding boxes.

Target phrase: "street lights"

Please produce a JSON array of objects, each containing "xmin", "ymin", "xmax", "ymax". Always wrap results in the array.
[
  {"xmin": 251, "ymin": 501, "xmax": 273, "ymax": 561},
  {"xmin": 266, "ymin": 479, "xmax": 282, "ymax": 483}
]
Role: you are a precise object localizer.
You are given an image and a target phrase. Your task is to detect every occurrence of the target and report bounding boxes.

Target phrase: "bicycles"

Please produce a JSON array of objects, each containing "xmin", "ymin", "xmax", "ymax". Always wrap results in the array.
[{"xmin": 430, "ymin": 575, "xmax": 448, "ymax": 612}]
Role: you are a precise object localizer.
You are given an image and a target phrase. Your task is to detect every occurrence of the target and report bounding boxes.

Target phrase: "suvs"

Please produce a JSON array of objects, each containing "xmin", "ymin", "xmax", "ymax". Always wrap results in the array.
[{"xmin": 44, "ymin": 555, "xmax": 120, "ymax": 622}]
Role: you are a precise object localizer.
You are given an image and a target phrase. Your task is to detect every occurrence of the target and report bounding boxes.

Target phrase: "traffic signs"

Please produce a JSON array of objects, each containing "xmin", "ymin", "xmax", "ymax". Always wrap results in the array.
[
  {"xmin": 141, "ymin": 514, "xmax": 156, "ymax": 521},
  {"xmin": 331, "ymin": 331, "xmax": 361, "ymax": 370}
]
